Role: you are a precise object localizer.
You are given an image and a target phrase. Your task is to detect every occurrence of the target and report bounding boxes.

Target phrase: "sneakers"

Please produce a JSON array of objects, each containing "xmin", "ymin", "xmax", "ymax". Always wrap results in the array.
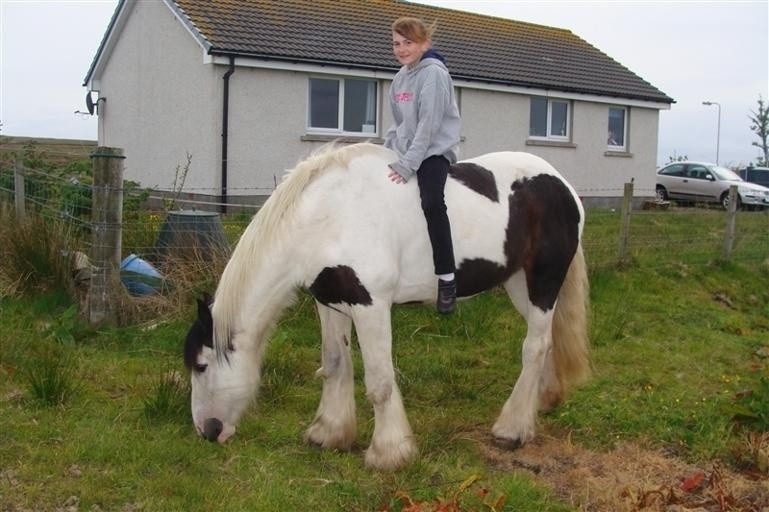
[{"xmin": 437, "ymin": 278, "xmax": 457, "ymax": 315}]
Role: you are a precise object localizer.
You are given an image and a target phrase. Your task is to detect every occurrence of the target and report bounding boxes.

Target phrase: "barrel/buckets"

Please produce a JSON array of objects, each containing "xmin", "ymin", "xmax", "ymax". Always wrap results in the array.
[
  {"xmin": 121, "ymin": 254, "xmax": 166, "ymax": 296},
  {"xmin": 152, "ymin": 209, "xmax": 227, "ymax": 268}
]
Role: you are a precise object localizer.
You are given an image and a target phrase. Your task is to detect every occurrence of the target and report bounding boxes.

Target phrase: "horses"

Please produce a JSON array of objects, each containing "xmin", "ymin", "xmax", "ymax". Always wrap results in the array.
[{"xmin": 183, "ymin": 138, "xmax": 593, "ymax": 475}]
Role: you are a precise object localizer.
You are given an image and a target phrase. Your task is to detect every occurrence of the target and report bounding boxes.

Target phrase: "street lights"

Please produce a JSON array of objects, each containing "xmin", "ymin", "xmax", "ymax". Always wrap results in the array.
[{"xmin": 702, "ymin": 101, "xmax": 721, "ymax": 165}]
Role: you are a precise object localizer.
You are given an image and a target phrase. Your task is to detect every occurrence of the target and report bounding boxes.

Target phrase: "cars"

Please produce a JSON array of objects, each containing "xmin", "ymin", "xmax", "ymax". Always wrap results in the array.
[{"xmin": 656, "ymin": 160, "xmax": 769, "ymax": 210}]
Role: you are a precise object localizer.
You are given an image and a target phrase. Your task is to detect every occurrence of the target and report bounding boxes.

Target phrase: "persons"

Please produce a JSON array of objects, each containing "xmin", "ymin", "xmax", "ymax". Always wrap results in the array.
[{"xmin": 381, "ymin": 19, "xmax": 463, "ymax": 315}]
